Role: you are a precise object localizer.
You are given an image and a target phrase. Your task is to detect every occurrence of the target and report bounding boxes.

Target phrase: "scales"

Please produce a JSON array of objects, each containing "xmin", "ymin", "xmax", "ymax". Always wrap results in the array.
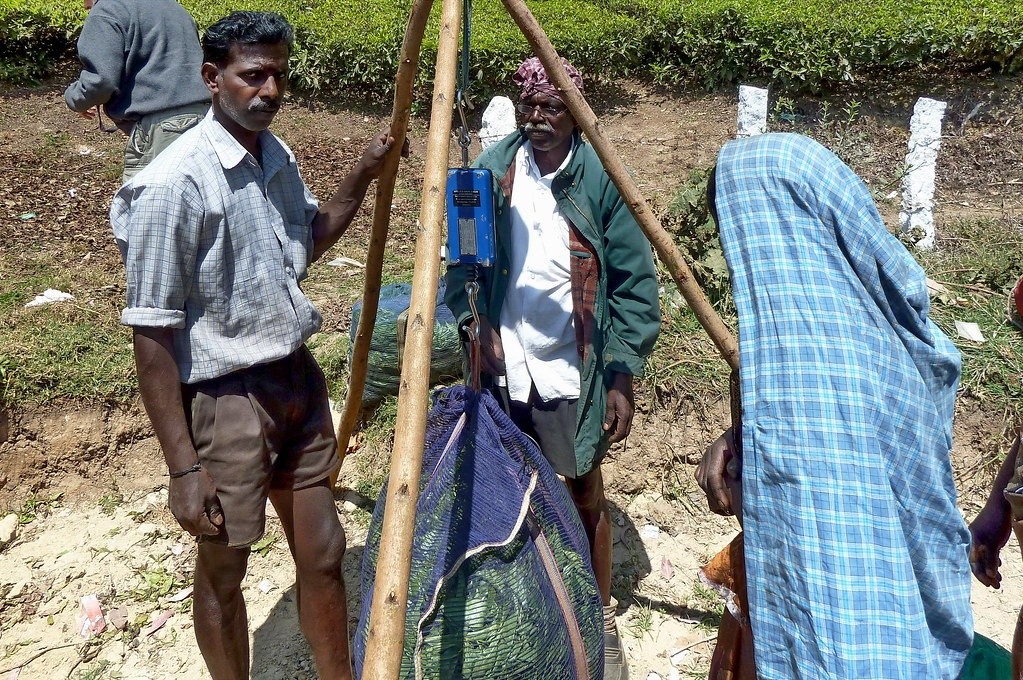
[{"xmin": 446, "ymin": 88, "xmax": 500, "ymax": 395}]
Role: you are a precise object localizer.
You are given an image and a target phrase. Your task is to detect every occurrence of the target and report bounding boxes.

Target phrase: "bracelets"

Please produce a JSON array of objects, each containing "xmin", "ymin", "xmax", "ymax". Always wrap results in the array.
[{"xmin": 168, "ymin": 461, "xmax": 200, "ymax": 477}]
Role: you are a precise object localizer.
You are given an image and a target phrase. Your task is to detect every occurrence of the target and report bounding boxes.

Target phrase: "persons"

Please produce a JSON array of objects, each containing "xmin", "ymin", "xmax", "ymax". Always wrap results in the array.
[
  {"xmin": 969, "ymin": 281, "xmax": 1022, "ymax": 680},
  {"xmin": 110, "ymin": 11, "xmax": 411, "ymax": 679},
  {"xmin": 443, "ymin": 58, "xmax": 661, "ymax": 680},
  {"xmin": 695, "ymin": 135, "xmax": 973, "ymax": 679},
  {"xmin": 64, "ymin": 0, "xmax": 211, "ymax": 187}
]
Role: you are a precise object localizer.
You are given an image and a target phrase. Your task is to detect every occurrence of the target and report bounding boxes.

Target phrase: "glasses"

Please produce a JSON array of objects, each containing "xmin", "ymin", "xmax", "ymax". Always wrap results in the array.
[{"xmin": 517, "ymin": 104, "xmax": 566, "ymax": 118}]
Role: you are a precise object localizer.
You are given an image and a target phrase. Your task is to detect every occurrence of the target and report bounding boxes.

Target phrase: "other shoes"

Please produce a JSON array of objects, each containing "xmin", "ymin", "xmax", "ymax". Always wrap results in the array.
[{"xmin": 600, "ymin": 627, "xmax": 627, "ymax": 680}]
